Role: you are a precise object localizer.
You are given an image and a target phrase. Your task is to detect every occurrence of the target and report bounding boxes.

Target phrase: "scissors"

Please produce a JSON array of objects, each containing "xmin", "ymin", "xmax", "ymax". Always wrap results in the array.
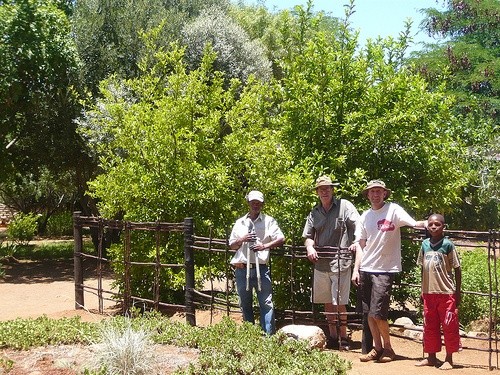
[{"xmin": 246, "ymin": 219, "xmax": 262, "ymax": 291}]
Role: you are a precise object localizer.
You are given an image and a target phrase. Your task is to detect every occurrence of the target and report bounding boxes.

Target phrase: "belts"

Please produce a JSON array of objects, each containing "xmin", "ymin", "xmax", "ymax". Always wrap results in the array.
[{"xmin": 243, "ymin": 264, "xmax": 266, "ymax": 269}]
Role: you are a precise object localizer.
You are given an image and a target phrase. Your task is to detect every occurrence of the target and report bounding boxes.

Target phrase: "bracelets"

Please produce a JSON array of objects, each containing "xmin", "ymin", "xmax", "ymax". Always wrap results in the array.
[
  {"xmin": 262, "ymin": 244, "xmax": 265, "ymax": 249},
  {"xmin": 235, "ymin": 239, "xmax": 242, "ymax": 246}
]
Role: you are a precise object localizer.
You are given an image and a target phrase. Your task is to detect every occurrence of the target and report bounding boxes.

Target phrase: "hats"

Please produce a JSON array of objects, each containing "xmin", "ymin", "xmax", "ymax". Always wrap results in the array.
[
  {"xmin": 361, "ymin": 180, "xmax": 391, "ymax": 200},
  {"xmin": 249, "ymin": 191, "xmax": 265, "ymax": 202},
  {"xmin": 311, "ymin": 177, "xmax": 340, "ymax": 189}
]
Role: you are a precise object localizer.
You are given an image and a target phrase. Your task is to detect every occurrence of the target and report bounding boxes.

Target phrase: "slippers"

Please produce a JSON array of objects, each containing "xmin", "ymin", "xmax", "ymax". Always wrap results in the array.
[
  {"xmin": 360, "ymin": 348, "xmax": 384, "ymax": 362},
  {"xmin": 379, "ymin": 348, "xmax": 395, "ymax": 362}
]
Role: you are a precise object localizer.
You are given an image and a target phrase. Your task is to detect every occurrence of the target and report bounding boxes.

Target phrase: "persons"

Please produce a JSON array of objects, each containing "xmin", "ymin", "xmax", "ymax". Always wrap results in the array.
[
  {"xmin": 415, "ymin": 214, "xmax": 464, "ymax": 369},
  {"xmin": 228, "ymin": 191, "xmax": 286, "ymax": 335},
  {"xmin": 352, "ymin": 180, "xmax": 429, "ymax": 362},
  {"xmin": 300, "ymin": 176, "xmax": 362, "ymax": 351}
]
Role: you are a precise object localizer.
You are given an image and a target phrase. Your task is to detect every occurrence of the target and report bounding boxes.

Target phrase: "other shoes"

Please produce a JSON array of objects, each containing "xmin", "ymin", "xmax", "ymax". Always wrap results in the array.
[
  {"xmin": 339, "ymin": 337, "xmax": 349, "ymax": 351},
  {"xmin": 325, "ymin": 336, "xmax": 339, "ymax": 349}
]
